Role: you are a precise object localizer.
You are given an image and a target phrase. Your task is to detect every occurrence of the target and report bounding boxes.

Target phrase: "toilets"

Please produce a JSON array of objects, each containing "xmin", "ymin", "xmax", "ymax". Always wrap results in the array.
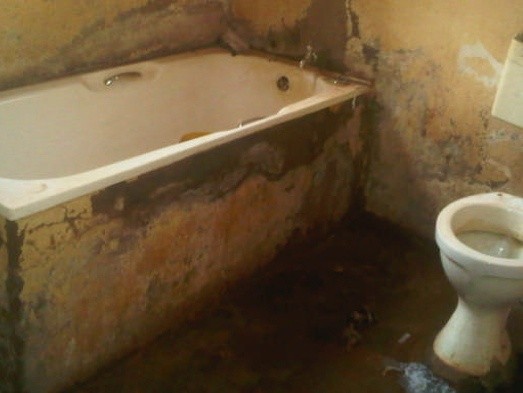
[{"xmin": 433, "ymin": 31, "xmax": 523, "ymax": 376}]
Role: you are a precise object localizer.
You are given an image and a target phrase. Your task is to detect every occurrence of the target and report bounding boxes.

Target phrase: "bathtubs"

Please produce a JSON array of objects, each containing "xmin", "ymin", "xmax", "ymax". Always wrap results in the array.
[{"xmin": 0, "ymin": 44, "xmax": 369, "ymax": 393}]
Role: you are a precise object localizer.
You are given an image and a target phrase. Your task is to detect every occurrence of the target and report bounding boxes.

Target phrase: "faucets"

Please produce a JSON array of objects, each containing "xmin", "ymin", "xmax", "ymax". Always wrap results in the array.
[{"xmin": 293, "ymin": 44, "xmax": 318, "ymax": 68}]
